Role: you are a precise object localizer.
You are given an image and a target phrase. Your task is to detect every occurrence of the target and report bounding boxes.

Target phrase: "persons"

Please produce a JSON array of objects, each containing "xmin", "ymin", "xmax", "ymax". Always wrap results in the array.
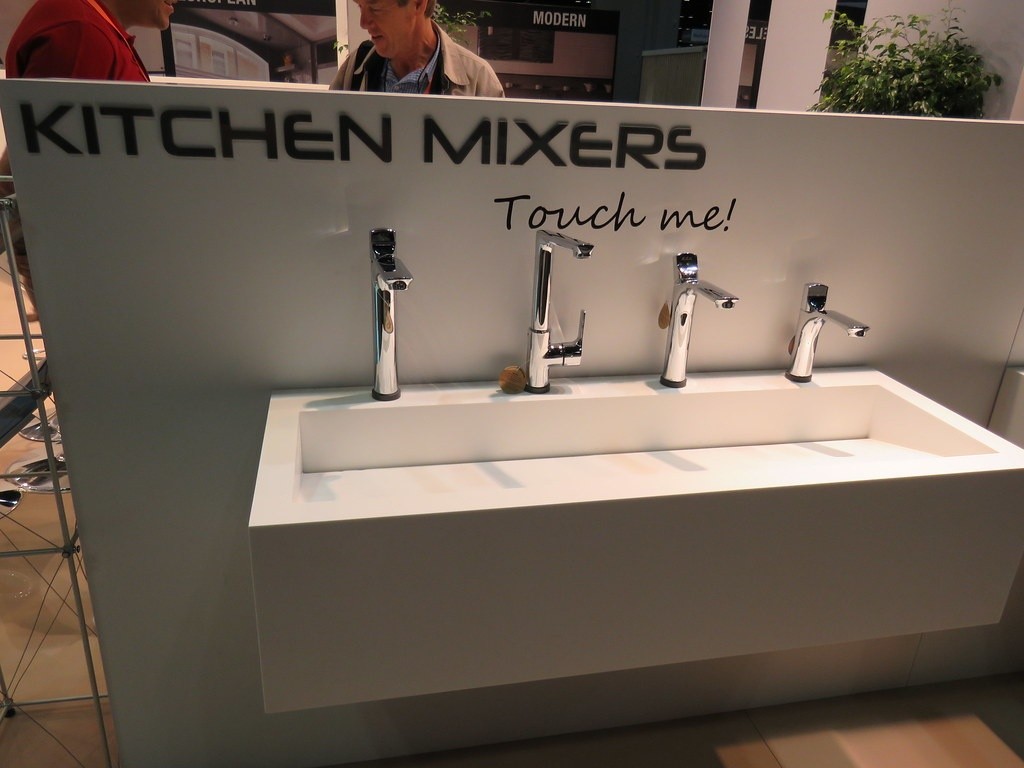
[
  {"xmin": 329, "ymin": 0, "xmax": 505, "ymax": 99},
  {"xmin": 5, "ymin": 0, "xmax": 177, "ymax": 83}
]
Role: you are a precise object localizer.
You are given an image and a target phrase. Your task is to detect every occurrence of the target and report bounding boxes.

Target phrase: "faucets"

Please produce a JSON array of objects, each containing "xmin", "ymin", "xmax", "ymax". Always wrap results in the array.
[
  {"xmin": 525, "ymin": 229, "xmax": 593, "ymax": 395},
  {"xmin": 656, "ymin": 253, "xmax": 738, "ymax": 389},
  {"xmin": 785, "ymin": 283, "xmax": 870, "ymax": 383},
  {"xmin": 369, "ymin": 226, "xmax": 413, "ymax": 401}
]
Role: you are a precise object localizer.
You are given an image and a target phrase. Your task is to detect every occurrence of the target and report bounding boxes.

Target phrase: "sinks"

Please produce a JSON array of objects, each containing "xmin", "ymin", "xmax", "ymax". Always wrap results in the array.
[{"xmin": 249, "ymin": 365, "xmax": 1024, "ymax": 714}]
